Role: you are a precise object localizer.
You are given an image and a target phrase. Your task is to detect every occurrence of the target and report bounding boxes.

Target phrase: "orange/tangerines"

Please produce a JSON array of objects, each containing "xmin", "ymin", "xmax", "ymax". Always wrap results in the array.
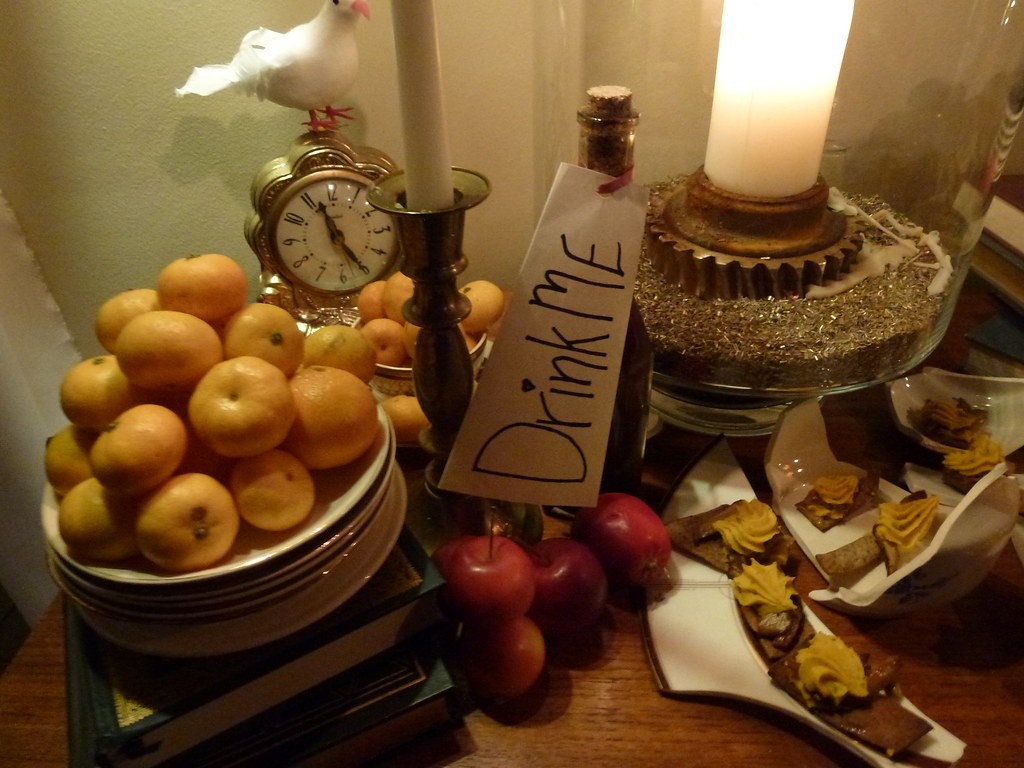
[{"xmin": 44, "ymin": 253, "xmax": 504, "ymax": 570}]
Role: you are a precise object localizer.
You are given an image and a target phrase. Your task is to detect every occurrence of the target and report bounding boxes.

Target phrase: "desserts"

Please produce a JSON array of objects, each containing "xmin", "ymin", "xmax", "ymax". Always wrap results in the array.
[
  {"xmin": 906, "ymin": 394, "xmax": 1024, "ymax": 518},
  {"xmin": 662, "ymin": 469, "xmax": 937, "ymax": 755}
]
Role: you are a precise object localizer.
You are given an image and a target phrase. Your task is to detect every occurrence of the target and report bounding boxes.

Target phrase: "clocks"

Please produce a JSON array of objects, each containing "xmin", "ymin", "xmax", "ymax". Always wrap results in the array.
[{"xmin": 243, "ymin": 139, "xmax": 404, "ymax": 308}]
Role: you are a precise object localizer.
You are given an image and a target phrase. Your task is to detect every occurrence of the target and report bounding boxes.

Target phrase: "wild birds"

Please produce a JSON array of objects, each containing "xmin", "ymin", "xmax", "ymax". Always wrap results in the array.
[{"xmin": 174, "ymin": 0, "xmax": 372, "ymax": 134}]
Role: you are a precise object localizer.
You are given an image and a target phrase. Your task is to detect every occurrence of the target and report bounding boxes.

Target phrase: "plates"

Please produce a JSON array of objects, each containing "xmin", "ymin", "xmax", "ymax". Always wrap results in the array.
[{"xmin": 43, "ymin": 402, "xmax": 407, "ymax": 656}]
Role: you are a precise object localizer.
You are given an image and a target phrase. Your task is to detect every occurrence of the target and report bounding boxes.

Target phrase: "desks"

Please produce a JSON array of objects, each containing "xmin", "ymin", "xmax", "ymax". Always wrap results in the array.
[{"xmin": 0, "ymin": 451, "xmax": 1023, "ymax": 767}]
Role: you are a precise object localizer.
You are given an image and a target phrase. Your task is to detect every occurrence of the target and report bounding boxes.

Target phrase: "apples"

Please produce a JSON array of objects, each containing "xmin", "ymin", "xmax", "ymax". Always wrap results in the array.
[{"xmin": 428, "ymin": 493, "xmax": 672, "ymax": 699}]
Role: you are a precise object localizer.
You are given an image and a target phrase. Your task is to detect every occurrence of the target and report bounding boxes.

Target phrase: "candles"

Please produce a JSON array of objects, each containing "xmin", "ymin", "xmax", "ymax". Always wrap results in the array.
[{"xmin": 703, "ymin": 0, "xmax": 856, "ymax": 198}]
